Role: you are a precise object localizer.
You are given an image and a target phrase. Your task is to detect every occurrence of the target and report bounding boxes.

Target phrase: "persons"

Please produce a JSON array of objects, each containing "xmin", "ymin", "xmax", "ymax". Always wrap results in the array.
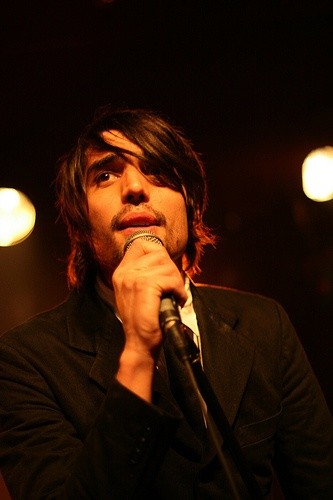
[{"xmin": 1, "ymin": 108, "xmax": 332, "ymax": 500}]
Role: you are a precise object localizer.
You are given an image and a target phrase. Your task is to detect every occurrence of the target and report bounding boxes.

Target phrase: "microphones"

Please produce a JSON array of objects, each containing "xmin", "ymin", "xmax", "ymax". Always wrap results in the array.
[{"xmin": 123, "ymin": 231, "xmax": 187, "ymax": 355}]
